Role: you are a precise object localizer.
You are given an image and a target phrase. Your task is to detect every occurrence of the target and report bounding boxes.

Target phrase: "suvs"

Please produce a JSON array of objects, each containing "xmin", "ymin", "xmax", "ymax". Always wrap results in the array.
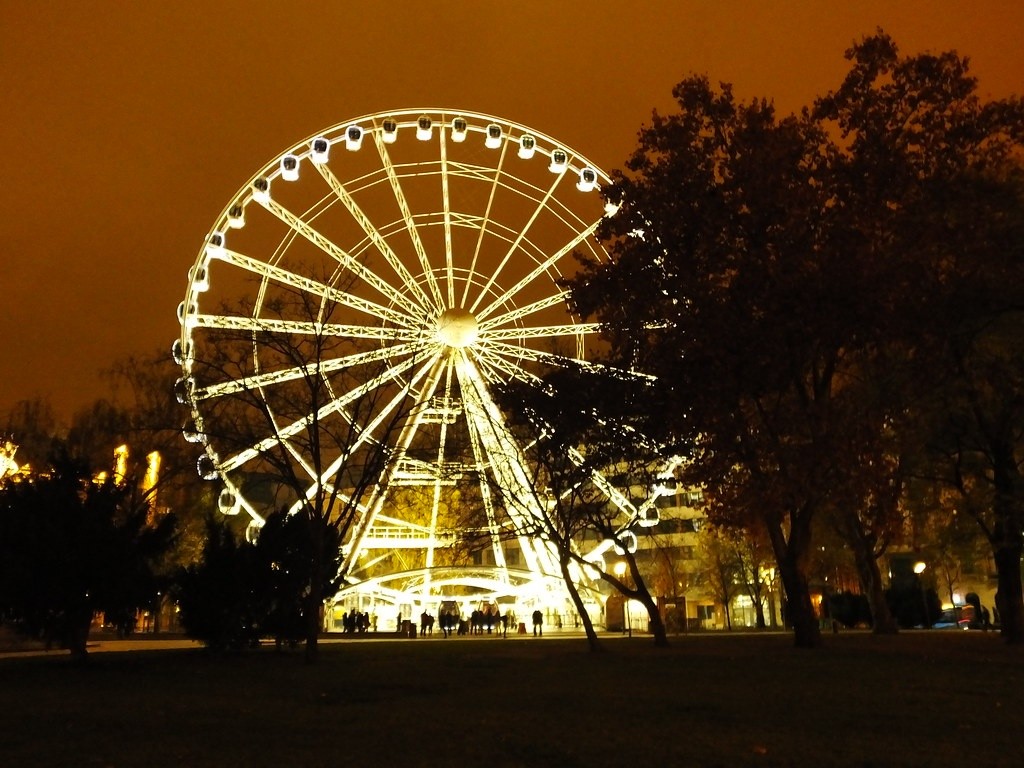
[{"xmin": 934, "ymin": 604, "xmax": 977, "ymax": 630}]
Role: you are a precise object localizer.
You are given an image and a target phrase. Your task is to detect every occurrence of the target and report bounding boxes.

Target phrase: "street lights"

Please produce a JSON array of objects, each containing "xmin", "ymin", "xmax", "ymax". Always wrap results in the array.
[{"xmin": 914, "ymin": 560, "xmax": 934, "ymax": 629}]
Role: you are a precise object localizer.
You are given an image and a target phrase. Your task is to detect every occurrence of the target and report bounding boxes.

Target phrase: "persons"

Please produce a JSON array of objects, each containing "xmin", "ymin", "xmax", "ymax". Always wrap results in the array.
[
  {"xmin": 419, "ymin": 610, "xmax": 507, "ymax": 638},
  {"xmin": 961, "ymin": 592, "xmax": 991, "ymax": 624},
  {"xmin": 532, "ymin": 610, "xmax": 544, "ymax": 635},
  {"xmin": 342, "ymin": 609, "xmax": 371, "ymax": 632}
]
